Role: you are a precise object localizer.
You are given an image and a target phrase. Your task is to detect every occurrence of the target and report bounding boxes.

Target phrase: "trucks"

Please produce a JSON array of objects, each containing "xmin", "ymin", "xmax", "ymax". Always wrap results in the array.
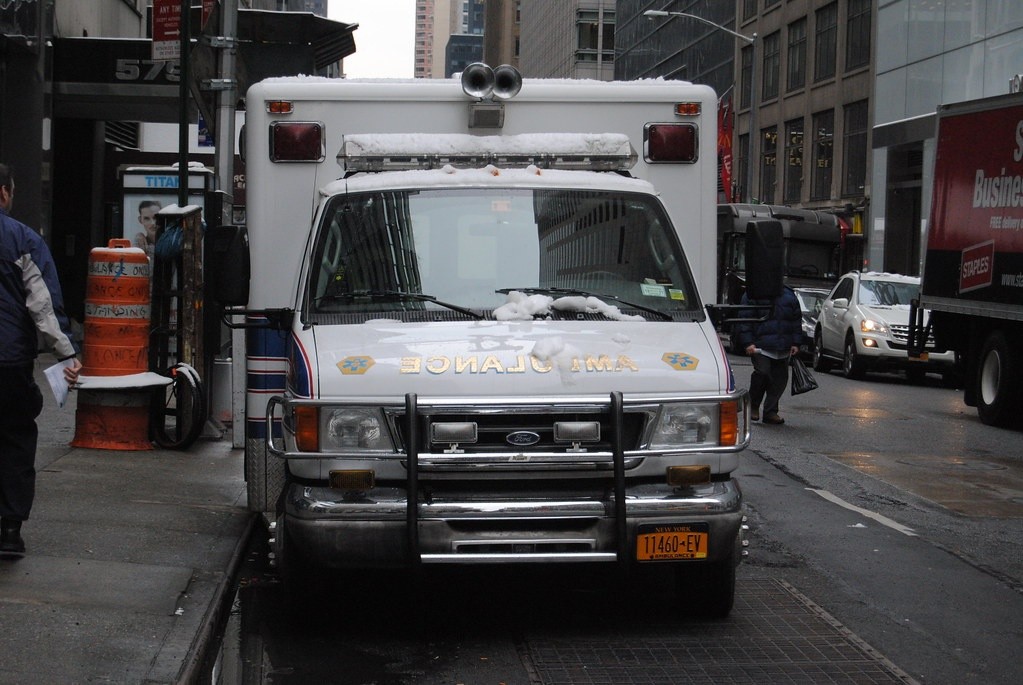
[
  {"xmin": 202, "ymin": 62, "xmax": 784, "ymax": 631},
  {"xmin": 717, "ymin": 203, "xmax": 841, "ymax": 355},
  {"xmin": 908, "ymin": 91, "xmax": 1023, "ymax": 427}
]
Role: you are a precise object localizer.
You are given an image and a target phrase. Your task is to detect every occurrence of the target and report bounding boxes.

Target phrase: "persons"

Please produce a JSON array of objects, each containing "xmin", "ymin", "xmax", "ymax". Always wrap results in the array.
[
  {"xmin": 132, "ymin": 200, "xmax": 161, "ymax": 290},
  {"xmin": 0, "ymin": 163, "xmax": 82, "ymax": 557},
  {"xmin": 737, "ymin": 285, "xmax": 803, "ymax": 424}
]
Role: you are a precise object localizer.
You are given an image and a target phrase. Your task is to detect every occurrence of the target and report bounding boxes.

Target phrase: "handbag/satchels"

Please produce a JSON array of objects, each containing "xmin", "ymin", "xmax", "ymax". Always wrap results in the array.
[{"xmin": 789, "ymin": 354, "xmax": 818, "ymax": 396}]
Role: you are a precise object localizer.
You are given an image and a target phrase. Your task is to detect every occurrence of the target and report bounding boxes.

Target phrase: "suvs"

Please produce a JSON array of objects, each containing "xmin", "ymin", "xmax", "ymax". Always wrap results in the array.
[{"xmin": 812, "ymin": 269, "xmax": 965, "ymax": 390}]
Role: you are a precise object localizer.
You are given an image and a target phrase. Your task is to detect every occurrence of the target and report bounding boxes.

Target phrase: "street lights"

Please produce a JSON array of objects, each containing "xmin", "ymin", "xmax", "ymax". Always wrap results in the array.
[{"xmin": 644, "ymin": 9, "xmax": 758, "ymax": 204}]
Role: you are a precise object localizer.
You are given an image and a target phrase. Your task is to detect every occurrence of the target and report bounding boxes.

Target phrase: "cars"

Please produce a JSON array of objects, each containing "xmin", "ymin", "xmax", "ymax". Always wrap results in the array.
[{"xmin": 789, "ymin": 287, "xmax": 843, "ymax": 370}]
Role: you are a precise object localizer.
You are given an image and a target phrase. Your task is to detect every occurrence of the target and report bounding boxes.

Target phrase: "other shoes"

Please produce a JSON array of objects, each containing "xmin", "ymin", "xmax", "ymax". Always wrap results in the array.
[
  {"xmin": 762, "ymin": 414, "xmax": 785, "ymax": 424},
  {"xmin": 0, "ymin": 527, "xmax": 25, "ymax": 556},
  {"xmin": 750, "ymin": 408, "xmax": 760, "ymax": 421}
]
